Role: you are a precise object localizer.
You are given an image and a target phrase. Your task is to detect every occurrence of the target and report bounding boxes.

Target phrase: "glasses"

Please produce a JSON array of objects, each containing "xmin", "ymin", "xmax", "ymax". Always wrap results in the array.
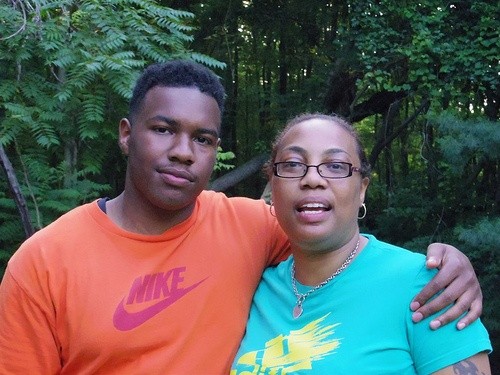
[{"xmin": 272, "ymin": 162, "xmax": 361, "ymax": 178}]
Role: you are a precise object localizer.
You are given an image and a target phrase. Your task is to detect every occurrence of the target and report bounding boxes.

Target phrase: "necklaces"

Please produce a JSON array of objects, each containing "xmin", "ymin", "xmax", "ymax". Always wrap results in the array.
[{"xmin": 291, "ymin": 235, "xmax": 360, "ymax": 318}]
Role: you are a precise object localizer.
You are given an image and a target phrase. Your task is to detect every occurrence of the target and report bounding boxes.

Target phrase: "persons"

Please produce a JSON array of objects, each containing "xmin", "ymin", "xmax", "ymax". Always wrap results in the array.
[
  {"xmin": 0, "ymin": 60, "xmax": 482, "ymax": 375},
  {"xmin": 229, "ymin": 112, "xmax": 493, "ymax": 375}
]
